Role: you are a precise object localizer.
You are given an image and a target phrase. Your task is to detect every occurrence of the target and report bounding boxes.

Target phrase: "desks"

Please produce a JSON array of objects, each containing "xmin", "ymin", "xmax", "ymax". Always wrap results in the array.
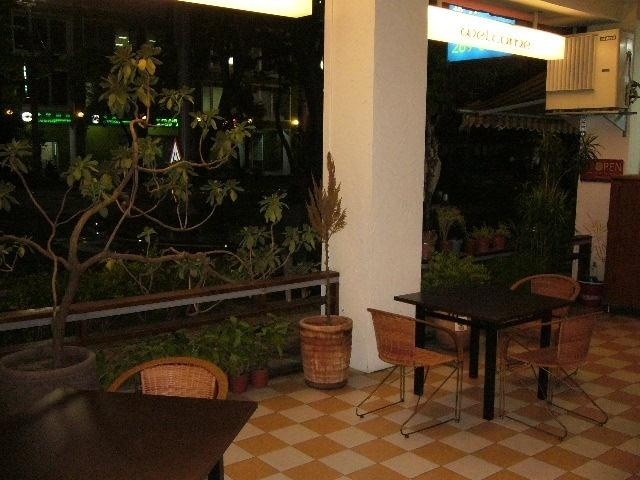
[
  {"xmin": 392, "ymin": 277, "xmax": 575, "ymax": 422},
  {"xmin": 2, "ymin": 384, "xmax": 262, "ymax": 480}
]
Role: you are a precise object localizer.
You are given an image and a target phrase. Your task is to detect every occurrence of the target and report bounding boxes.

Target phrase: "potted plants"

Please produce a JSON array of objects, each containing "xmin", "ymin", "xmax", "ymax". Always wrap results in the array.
[
  {"xmin": 298, "ymin": 151, "xmax": 356, "ymax": 395},
  {"xmin": 228, "ymin": 309, "xmax": 293, "ymax": 394},
  {"xmin": 422, "ymin": 204, "xmax": 514, "ymax": 266}
]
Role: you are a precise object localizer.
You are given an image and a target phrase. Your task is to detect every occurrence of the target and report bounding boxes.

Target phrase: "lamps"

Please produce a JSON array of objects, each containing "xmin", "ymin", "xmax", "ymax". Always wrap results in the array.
[{"xmin": 426, "ymin": 0, "xmax": 568, "ymax": 62}]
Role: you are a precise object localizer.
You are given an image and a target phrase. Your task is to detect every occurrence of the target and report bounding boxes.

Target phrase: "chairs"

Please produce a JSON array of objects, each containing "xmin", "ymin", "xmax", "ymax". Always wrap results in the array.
[
  {"xmin": 499, "ymin": 308, "xmax": 610, "ymax": 445},
  {"xmin": 102, "ymin": 353, "xmax": 236, "ymax": 403},
  {"xmin": 352, "ymin": 308, "xmax": 467, "ymax": 439},
  {"xmin": 502, "ymin": 266, "xmax": 581, "ymax": 381}
]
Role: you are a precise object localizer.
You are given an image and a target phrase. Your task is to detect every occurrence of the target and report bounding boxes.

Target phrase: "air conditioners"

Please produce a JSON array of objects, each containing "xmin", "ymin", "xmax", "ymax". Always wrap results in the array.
[{"xmin": 543, "ymin": 27, "xmax": 635, "ymax": 118}]
